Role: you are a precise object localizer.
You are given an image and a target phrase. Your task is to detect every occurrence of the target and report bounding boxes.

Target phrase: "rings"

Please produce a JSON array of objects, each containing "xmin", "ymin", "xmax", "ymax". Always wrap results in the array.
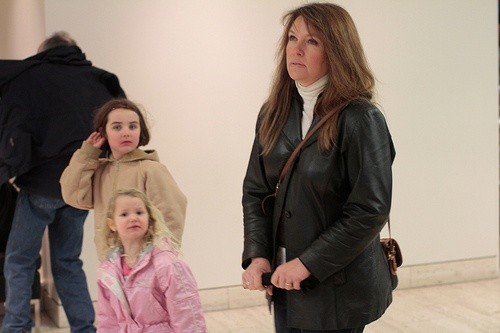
[
  {"xmin": 285, "ymin": 283, "xmax": 292, "ymax": 287},
  {"xmin": 245, "ymin": 281, "xmax": 250, "ymax": 286}
]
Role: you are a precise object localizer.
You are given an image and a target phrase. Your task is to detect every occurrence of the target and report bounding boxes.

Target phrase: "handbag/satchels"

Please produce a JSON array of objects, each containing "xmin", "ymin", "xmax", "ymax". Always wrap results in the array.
[{"xmin": 381, "ymin": 238, "xmax": 403, "ymax": 290}]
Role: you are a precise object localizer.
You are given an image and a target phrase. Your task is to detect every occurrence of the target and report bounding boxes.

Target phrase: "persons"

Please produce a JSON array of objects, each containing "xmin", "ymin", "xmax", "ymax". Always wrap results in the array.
[
  {"xmin": 0, "ymin": 31, "xmax": 128, "ymax": 333},
  {"xmin": 59, "ymin": 98, "xmax": 188, "ymax": 262},
  {"xmin": 241, "ymin": 3, "xmax": 396, "ymax": 333},
  {"xmin": 92, "ymin": 187, "xmax": 208, "ymax": 333}
]
